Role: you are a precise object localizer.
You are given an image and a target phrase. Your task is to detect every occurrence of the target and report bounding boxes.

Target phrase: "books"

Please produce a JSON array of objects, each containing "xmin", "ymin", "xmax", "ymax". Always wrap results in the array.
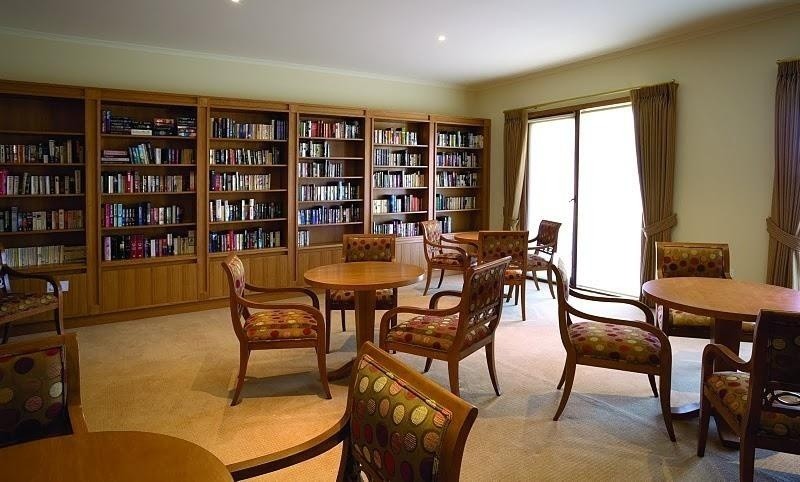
[
  {"xmin": 374, "ymin": 127, "xmax": 425, "ymax": 238},
  {"xmin": 209, "ymin": 117, "xmax": 287, "ymax": 251},
  {"xmin": 99, "ymin": 110, "xmax": 196, "ymax": 260},
  {"xmin": 298, "ymin": 230, "xmax": 309, "ymax": 247},
  {"xmin": 299, "ymin": 120, "xmax": 361, "ymax": 225},
  {"xmin": 0, "ymin": 139, "xmax": 87, "ymax": 269},
  {"xmin": 437, "ymin": 131, "xmax": 482, "ymax": 233}
]
